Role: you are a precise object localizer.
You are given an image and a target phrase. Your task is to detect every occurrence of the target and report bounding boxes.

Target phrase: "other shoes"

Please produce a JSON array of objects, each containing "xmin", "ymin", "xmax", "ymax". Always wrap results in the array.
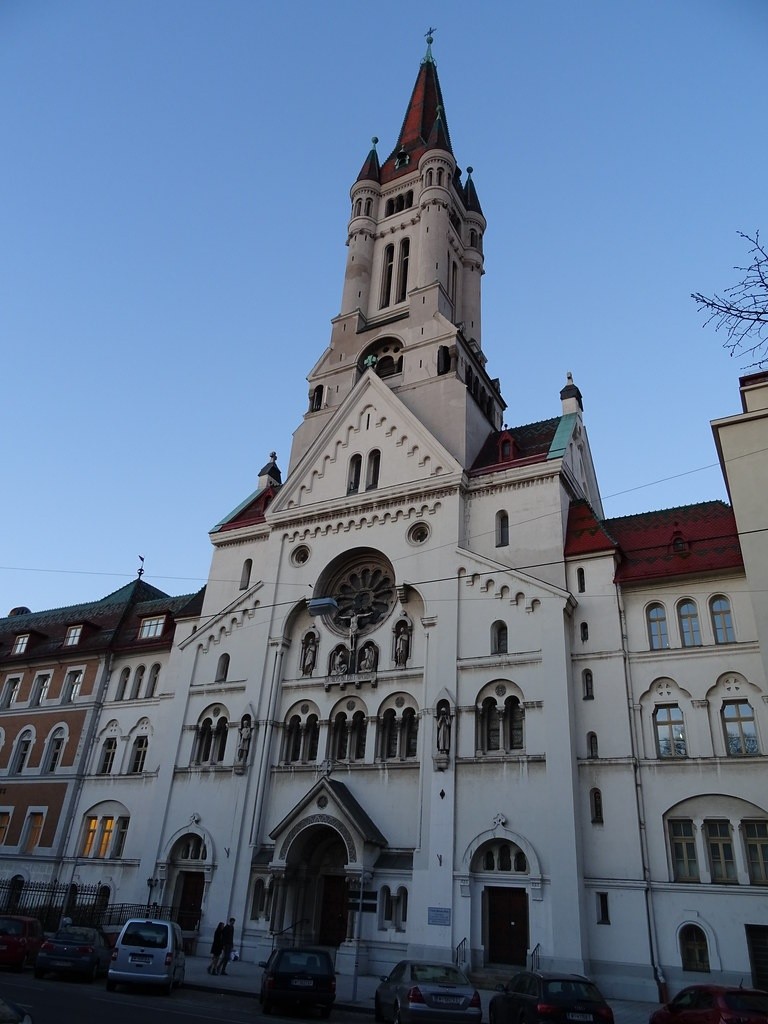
[
  {"xmin": 211, "ymin": 971, "xmax": 218, "ymax": 975},
  {"xmin": 207, "ymin": 967, "xmax": 211, "ymax": 974},
  {"xmin": 221, "ymin": 971, "xmax": 228, "ymax": 975},
  {"xmin": 216, "ymin": 967, "xmax": 220, "ymax": 973}
]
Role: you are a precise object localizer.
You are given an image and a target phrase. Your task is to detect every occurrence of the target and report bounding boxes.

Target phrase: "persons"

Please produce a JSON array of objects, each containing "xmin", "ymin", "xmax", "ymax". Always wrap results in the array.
[
  {"xmin": 305, "ymin": 638, "xmax": 315, "ymax": 674},
  {"xmin": 396, "ymin": 626, "xmax": 408, "ymax": 666},
  {"xmin": 207, "ymin": 922, "xmax": 224, "ymax": 975},
  {"xmin": 366, "ymin": 646, "xmax": 375, "ymax": 671},
  {"xmin": 217, "ymin": 918, "xmax": 235, "ymax": 975},
  {"xmin": 338, "ymin": 610, "xmax": 373, "ymax": 638},
  {"xmin": 438, "ymin": 707, "xmax": 451, "ymax": 754},
  {"xmin": 336, "ymin": 651, "xmax": 347, "ymax": 675},
  {"xmin": 238, "ymin": 720, "xmax": 251, "ymax": 762}
]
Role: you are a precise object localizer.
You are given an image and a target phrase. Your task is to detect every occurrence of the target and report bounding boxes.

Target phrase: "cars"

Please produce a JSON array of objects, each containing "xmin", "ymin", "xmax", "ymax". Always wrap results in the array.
[
  {"xmin": 375, "ymin": 960, "xmax": 483, "ymax": 1024},
  {"xmin": 34, "ymin": 926, "xmax": 115, "ymax": 985},
  {"xmin": 650, "ymin": 986, "xmax": 768, "ymax": 1024},
  {"xmin": 0, "ymin": 915, "xmax": 46, "ymax": 973}
]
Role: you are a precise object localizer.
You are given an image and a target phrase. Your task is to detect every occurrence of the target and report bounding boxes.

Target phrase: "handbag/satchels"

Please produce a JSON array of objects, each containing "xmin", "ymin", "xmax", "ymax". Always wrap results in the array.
[{"xmin": 230, "ymin": 949, "xmax": 240, "ymax": 961}]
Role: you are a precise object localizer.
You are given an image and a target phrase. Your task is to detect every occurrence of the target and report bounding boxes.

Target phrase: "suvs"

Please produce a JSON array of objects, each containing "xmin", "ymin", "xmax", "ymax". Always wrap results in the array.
[
  {"xmin": 489, "ymin": 972, "xmax": 615, "ymax": 1024},
  {"xmin": 258, "ymin": 947, "xmax": 339, "ymax": 1018}
]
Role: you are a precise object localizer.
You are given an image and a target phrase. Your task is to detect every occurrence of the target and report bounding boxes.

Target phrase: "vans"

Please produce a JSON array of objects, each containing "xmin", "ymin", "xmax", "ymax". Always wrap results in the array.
[{"xmin": 106, "ymin": 918, "xmax": 186, "ymax": 996}]
